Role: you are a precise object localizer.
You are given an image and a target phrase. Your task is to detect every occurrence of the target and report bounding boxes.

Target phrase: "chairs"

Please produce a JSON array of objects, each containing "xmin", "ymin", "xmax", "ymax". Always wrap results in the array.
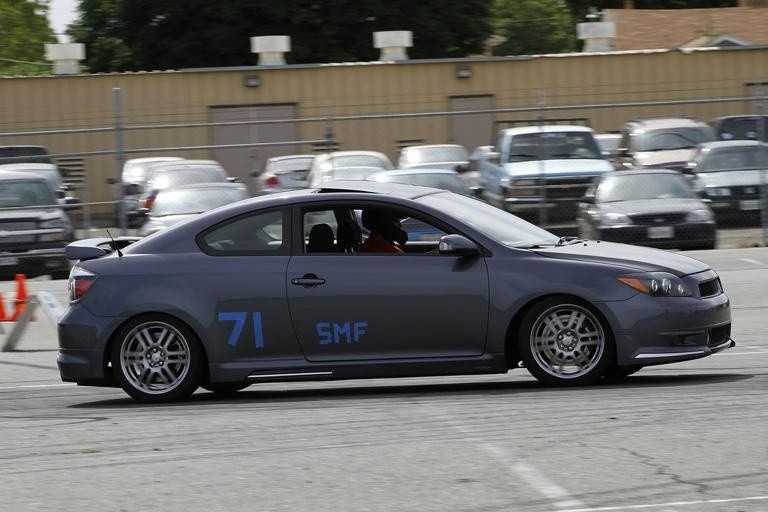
[{"xmin": 308, "ymin": 220, "xmax": 361, "ymax": 252}]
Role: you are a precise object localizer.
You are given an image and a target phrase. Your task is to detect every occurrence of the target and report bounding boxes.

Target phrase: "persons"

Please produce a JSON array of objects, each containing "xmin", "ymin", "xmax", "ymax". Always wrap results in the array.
[{"xmin": 354, "ymin": 204, "xmax": 411, "ymax": 256}]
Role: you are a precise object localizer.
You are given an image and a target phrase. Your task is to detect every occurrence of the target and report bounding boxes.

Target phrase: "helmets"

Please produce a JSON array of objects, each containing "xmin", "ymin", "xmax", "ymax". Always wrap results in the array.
[{"xmin": 360, "ymin": 205, "xmax": 411, "ymax": 246}]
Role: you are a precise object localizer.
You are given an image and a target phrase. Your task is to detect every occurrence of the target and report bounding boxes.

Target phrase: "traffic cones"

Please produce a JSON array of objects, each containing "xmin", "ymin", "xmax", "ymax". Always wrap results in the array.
[{"xmin": 0, "ymin": 273, "xmax": 38, "ymax": 322}]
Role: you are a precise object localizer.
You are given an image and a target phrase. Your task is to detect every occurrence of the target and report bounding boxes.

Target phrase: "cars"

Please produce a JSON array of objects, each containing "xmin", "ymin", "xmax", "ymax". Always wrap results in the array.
[
  {"xmin": 715, "ymin": 112, "xmax": 768, "ymax": 141},
  {"xmin": 57, "ymin": 183, "xmax": 733, "ymax": 406},
  {"xmin": 249, "ymin": 144, "xmax": 473, "ymax": 201},
  {"xmin": 682, "ymin": 139, "xmax": 766, "ymax": 211},
  {"xmin": 576, "ymin": 169, "xmax": 717, "ymax": 250},
  {"xmin": 106, "ymin": 156, "xmax": 251, "ymax": 237}
]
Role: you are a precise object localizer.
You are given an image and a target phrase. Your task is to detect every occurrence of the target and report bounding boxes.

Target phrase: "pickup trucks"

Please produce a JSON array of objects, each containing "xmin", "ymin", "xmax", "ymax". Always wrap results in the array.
[{"xmin": 478, "ymin": 126, "xmax": 617, "ymax": 222}]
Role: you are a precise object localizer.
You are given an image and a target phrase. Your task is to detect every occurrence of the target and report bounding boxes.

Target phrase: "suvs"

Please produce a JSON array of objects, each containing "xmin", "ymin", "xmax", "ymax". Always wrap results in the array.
[
  {"xmin": 619, "ymin": 118, "xmax": 720, "ymax": 174},
  {"xmin": 0, "ymin": 145, "xmax": 84, "ymax": 280}
]
